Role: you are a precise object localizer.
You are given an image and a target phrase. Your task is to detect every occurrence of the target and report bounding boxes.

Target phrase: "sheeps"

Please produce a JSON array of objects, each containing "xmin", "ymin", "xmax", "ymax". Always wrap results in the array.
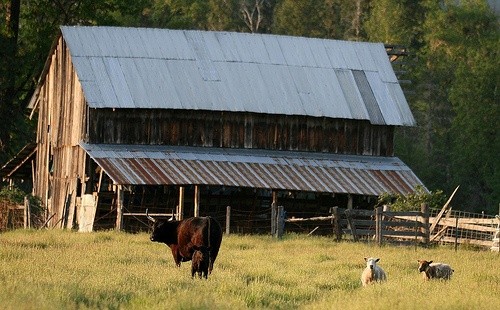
[
  {"xmin": 417, "ymin": 259, "xmax": 454, "ymax": 282},
  {"xmin": 361, "ymin": 257, "xmax": 387, "ymax": 287}
]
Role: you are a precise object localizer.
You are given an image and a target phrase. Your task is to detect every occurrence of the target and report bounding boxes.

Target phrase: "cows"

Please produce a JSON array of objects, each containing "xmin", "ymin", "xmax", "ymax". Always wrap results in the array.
[{"xmin": 144, "ymin": 208, "xmax": 223, "ymax": 281}]
